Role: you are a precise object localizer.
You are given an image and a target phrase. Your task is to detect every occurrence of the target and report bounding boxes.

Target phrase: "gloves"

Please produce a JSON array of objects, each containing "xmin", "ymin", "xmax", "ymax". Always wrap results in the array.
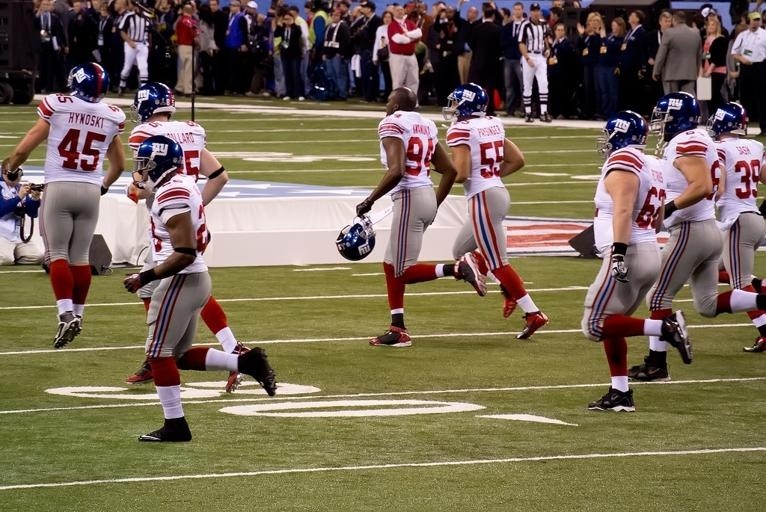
[
  {"xmin": 610, "ymin": 244, "xmax": 633, "ymax": 285},
  {"xmin": 355, "ymin": 197, "xmax": 374, "ymax": 219},
  {"xmin": 7, "ymin": 168, "xmax": 23, "ymax": 182},
  {"xmin": 122, "ymin": 267, "xmax": 158, "ymax": 295}
]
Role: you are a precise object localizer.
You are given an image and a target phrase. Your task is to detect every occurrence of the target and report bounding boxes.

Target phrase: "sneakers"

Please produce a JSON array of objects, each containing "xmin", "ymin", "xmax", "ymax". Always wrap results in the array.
[
  {"xmin": 117, "ymin": 81, "xmax": 128, "ymax": 97},
  {"xmin": 126, "ymin": 359, "xmax": 158, "ymax": 387},
  {"xmin": 742, "ymin": 337, "xmax": 765, "ymax": 353},
  {"xmin": 368, "ymin": 330, "xmax": 416, "ymax": 349},
  {"xmin": 173, "ymin": 85, "xmax": 349, "ymax": 104},
  {"xmin": 140, "ymin": 420, "xmax": 196, "ymax": 447},
  {"xmin": 499, "ymin": 107, "xmax": 554, "ymax": 126},
  {"xmin": 585, "ymin": 387, "xmax": 637, "ymax": 414},
  {"xmin": 53, "ymin": 316, "xmax": 83, "ymax": 348},
  {"xmin": 628, "ymin": 354, "xmax": 671, "ymax": 383},
  {"xmin": 226, "ymin": 346, "xmax": 276, "ymax": 397},
  {"xmin": 500, "ymin": 284, "xmax": 550, "ymax": 340},
  {"xmin": 465, "ymin": 250, "xmax": 489, "ymax": 298},
  {"xmin": 662, "ymin": 309, "xmax": 697, "ymax": 365}
]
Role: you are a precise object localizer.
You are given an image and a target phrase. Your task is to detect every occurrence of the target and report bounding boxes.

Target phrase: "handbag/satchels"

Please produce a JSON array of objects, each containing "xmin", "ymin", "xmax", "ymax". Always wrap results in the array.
[{"xmin": 696, "ymin": 75, "xmax": 713, "ymax": 101}]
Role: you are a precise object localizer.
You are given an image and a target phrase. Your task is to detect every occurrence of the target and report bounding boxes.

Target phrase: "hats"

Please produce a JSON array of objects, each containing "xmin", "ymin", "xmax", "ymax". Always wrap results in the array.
[
  {"xmin": 527, "ymin": 3, "xmax": 542, "ymax": 13},
  {"xmin": 748, "ymin": 12, "xmax": 761, "ymax": 20},
  {"xmin": 230, "ymin": 2, "xmax": 259, "ymax": 10}
]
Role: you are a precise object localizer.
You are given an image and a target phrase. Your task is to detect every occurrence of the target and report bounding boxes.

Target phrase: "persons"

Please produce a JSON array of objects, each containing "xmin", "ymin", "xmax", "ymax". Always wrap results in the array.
[
  {"xmin": 123, "ymin": 135, "xmax": 276, "ymax": 441},
  {"xmin": 125, "ymin": 81, "xmax": 251, "ymax": 392},
  {"xmin": 356, "ymin": 87, "xmax": 488, "ymax": 347},
  {"xmin": 627, "ymin": 91, "xmax": 766, "ymax": 382},
  {"xmin": 2, "ymin": 62, "xmax": 127, "ymax": 348},
  {"xmin": 1, "ymin": 159, "xmax": 44, "ymax": 266},
  {"xmin": 582, "ymin": 110, "xmax": 693, "ymax": 411},
  {"xmin": 442, "ymin": 83, "xmax": 549, "ymax": 340},
  {"xmin": 706, "ymin": 102, "xmax": 766, "ymax": 354}
]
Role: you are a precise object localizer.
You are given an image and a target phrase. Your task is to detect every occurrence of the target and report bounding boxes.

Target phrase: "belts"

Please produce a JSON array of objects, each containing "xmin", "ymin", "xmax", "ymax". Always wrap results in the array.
[{"xmin": 526, "ymin": 49, "xmax": 544, "ymax": 55}]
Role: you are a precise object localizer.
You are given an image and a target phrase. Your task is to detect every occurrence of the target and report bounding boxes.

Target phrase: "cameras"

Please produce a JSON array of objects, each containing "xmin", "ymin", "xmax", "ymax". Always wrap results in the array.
[{"xmin": 29, "ymin": 183, "xmax": 45, "ymax": 192}]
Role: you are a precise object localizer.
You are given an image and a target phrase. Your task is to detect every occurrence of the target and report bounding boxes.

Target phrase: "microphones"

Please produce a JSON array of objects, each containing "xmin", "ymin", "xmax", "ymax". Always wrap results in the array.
[
  {"xmin": 131, "ymin": 0, "xmax": 152, "ymax": 14},
  {"xmin": 182, "ymin": 19, "xmax": 205, "ymax": 33}
]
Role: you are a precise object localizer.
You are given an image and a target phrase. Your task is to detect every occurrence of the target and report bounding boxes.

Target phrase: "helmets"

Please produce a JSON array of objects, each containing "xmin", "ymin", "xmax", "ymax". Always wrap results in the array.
[
  {"xmin": 134, "ymin": 81, "xmax": 178, "ymax": 122},
  {"xmin": 335, "ymin": 215, "xmax": 376, "ymax": 262},
  {"xmin": 711, "ymin": 101, "xmax": 749, "ymax": 136},
  {"xmin": 66, "ymin": 61, "xmax": 112, "ymax": 104},
  {"xmin": 655, "ymin": 94, "xmax": 698, "ymax": 143},
  {"xmin": 137, "ymin": 135, "xmax": 183, "ymax": 185},
  {"xmin": 449, "ymin": 82, "xmax": 488, "ymax": 117},
  {"xmin": 604, "ymin": 110, "xmax": 647, "ymax": 153}
]
